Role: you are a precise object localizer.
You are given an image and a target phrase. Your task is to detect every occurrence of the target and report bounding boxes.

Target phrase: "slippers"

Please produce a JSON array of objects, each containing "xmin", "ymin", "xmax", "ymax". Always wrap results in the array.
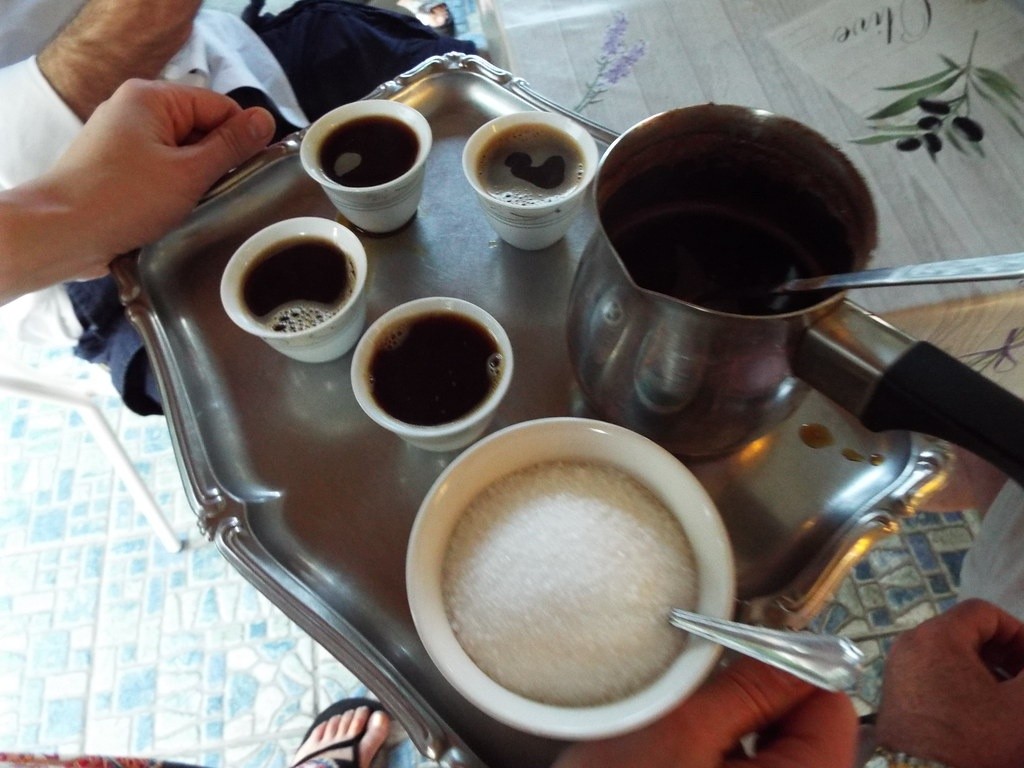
[{"xmin": 289, "ymin": 697, "xmax": 389, "ymax": 768}]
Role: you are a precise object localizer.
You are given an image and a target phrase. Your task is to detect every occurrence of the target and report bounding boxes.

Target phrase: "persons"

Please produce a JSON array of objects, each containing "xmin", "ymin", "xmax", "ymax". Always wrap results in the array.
[
  {"xmin": 0, "ymin": 0, "xmax": 493, "ymax": 417},
  {"xmin": 0, "ymin": 73, "xmax": 401, "ymax": 768}
]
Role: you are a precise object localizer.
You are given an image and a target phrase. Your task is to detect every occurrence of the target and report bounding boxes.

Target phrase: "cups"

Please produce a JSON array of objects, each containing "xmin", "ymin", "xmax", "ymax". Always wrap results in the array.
[
  {"xmin": 349, "ymin": 296, "xmax": 515, "ymax": 453},
  {"xmin": 220, "ymin": 216, "xmax": 368, "ymax": 365},
  {"xmin": 299, "ymin": 98, "xmax": 433, "ymax": 234},
  {"xmin": 461, "ymin": 109, "xmax": 599, "ymax": 252}
]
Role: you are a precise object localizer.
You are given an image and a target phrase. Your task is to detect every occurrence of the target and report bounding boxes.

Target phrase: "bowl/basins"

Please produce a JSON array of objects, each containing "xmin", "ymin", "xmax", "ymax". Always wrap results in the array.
[{"xmin": 404, "ymin": 416, "xmax": 736, "ymax": 742}]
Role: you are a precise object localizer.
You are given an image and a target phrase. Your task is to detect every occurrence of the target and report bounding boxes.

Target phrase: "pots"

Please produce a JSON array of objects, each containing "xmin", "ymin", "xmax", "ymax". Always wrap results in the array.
[{"xmin": 564, "ymin": 100, "xmax": 1024, "ymax": 491}]
